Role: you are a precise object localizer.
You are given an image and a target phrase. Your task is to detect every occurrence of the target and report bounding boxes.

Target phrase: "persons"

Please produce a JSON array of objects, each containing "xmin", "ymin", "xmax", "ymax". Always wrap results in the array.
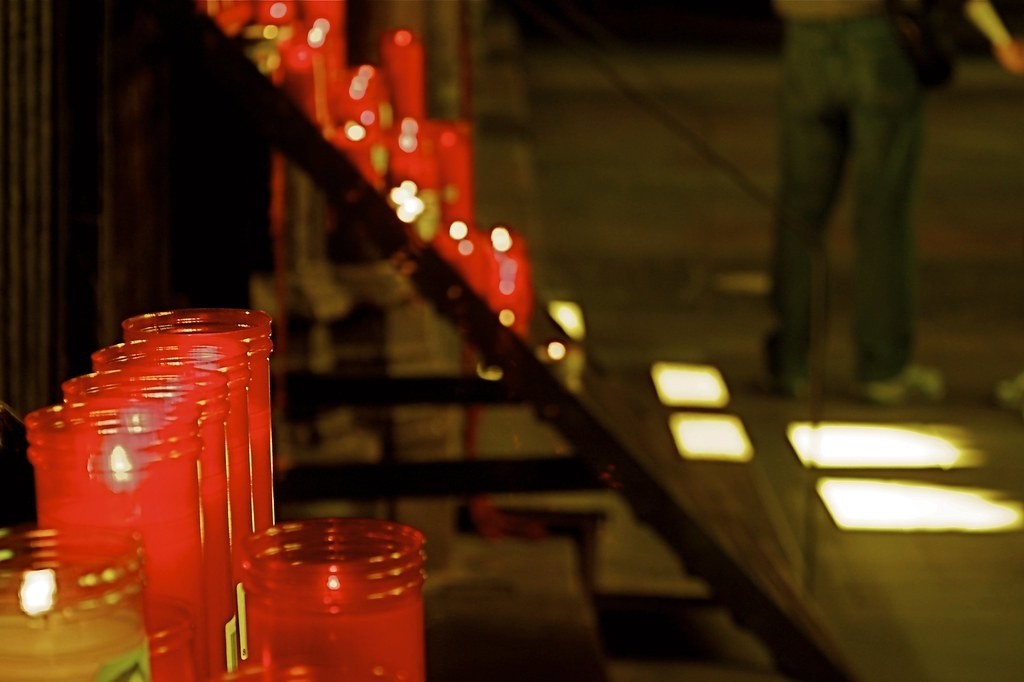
[
  {"xmin": 739, "ymin": 0, "xmax": 951, "ymax": 402},
  {"xmin": 965, "ymin": 0, "xmax": 1024, "ymax": 410}
]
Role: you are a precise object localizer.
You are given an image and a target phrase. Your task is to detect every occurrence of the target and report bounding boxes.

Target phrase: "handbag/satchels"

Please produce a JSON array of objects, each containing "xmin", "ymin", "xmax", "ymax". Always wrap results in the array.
[{"xmin": 882, "ymin": 0, "xmax": 960, "ymax": 86}]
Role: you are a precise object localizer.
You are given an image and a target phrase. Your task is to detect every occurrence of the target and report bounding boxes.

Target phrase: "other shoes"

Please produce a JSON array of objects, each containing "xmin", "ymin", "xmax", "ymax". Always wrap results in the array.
[
  {"xmin": 751, "ymin": 366, "xmax": 813, "ymax": 402},
  {"xmin": 847, "ymin": 380, "xmax": 905, "ymax": 405}
]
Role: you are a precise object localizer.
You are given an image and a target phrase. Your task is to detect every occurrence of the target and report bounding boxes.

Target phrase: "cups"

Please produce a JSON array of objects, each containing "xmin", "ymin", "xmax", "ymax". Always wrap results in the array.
[{"xmin": 0, "ymin": 308, "xmax": 425, "ymax": 682}]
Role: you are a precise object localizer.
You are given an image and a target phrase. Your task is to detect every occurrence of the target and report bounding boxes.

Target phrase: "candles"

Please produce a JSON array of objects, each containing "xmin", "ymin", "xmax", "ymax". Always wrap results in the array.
[{"xmin": 0, "ymin": 0, "xmax": 534, "ymax": 682}]
